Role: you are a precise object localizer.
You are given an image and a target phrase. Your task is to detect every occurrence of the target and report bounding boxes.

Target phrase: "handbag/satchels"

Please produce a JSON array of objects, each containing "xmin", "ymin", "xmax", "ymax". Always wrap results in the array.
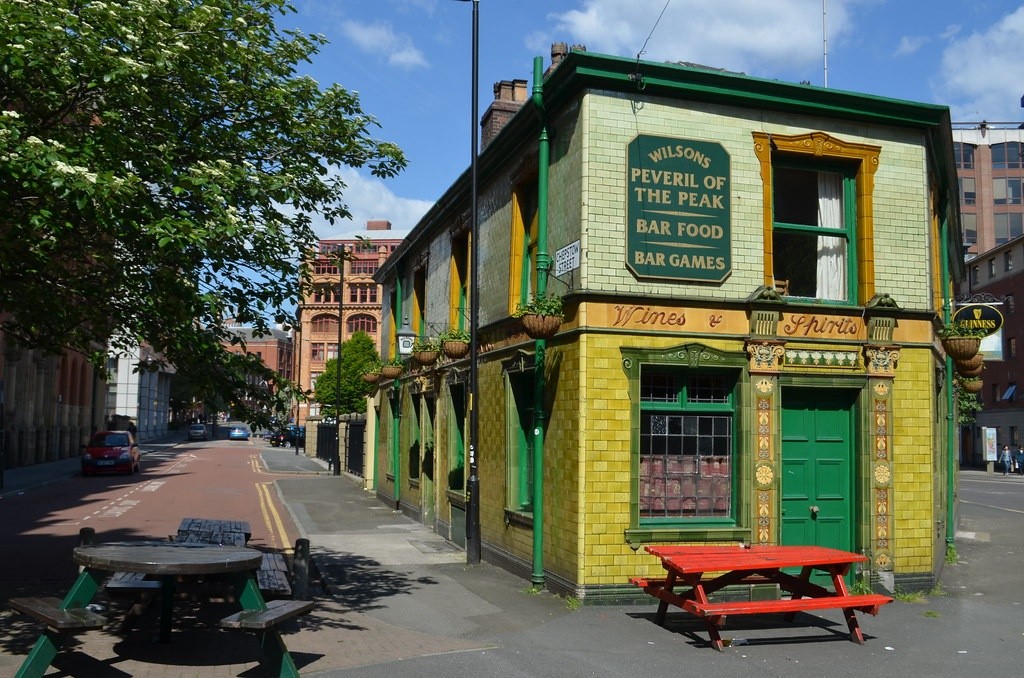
[
  {"xmin": 1011, "ymin": 465, "xmax": 1014, "ymax": 472},
  {"xmin": 1015, "ymin": 462, "xmax": 1018, "ymax": 468}
]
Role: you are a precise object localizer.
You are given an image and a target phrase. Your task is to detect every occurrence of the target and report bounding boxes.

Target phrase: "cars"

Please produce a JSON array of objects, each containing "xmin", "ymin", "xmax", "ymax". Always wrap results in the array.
[
  {"xmin": 79, "ymin": 430, "xmax": 141, "ymax": 477},
  {"xmin": 188, "ymin": 424, "xmax": 208, "ymax": 441},
  {"xmin": 229, "ymin": 425, "xmax": 248, "ymax": 440}
]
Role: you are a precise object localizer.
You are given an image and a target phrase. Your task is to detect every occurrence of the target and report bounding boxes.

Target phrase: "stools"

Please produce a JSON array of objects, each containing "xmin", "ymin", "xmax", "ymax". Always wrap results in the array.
[
  {"xmin": 219, "ymin": 599, "xmax": 326, "ymax": 678},
  {"xmin": 12, "ymin": 596, "xmax": 109, "ymax": 678}
]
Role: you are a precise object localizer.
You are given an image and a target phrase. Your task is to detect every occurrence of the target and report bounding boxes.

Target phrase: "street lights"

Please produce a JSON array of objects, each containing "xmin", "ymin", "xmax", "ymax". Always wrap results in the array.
[
  {"xmin": 296, "ymin": 322, "xmax": 303, "ymax": 455},
  {"xmin": 333, "ymin": 243, "xmax": 346, "ymax": 475}
]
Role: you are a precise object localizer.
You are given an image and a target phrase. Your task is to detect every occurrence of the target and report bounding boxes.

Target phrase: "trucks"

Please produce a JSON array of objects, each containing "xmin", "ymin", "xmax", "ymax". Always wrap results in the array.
[{"xmin": 271, "ymin": 425, "xmax": 306, "ymax": 447}]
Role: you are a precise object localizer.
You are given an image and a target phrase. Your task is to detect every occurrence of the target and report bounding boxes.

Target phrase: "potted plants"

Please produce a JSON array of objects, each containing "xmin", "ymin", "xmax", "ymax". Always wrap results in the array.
[
  {"xmin": 958, "ymin": 376, "xmax": 983, "ymax": 392},
  {"xmin": 379, "ymin": 356, "xmax": 405, "ymax": 379},
  {"xmin": 438, "ymin": 326, "xmax": 472, "ymax": 359},
  {"xmin": 411, "ymin": 337, "xmax": 439, "ymax": 366},
  {"xmin": 512, "ymin": 290, "xmax": 566, "ymax": 339},
  {"xmin": 939, "ymin": 321, "xmax": 988, "ymax": 360},
  {"xmin": 361, "ymin": 362, "xmax": 381, "ymax": 384}
]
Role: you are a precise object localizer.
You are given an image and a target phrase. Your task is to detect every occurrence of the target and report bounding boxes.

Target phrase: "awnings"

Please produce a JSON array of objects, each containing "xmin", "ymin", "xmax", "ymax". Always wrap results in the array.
[
  {"xmin": 1001, "ymin": 385, "xmax": 1016, "ymax": 400},
  {"xmin": 159, "ymin": 370, "xmax": 175, "ymax": 375}
]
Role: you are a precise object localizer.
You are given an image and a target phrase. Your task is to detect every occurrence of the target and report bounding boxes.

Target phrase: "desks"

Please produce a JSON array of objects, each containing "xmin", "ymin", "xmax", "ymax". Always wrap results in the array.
[
  {"xmin": 12, "ymin": 545, "xmax": 301, "ymax": 678},
  {"xmin": 168, "ymin": 520, "xmax": 251, "ymax": 549}
]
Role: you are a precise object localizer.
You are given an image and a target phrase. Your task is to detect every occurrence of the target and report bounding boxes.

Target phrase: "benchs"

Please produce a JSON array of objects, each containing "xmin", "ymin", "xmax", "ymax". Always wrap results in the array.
[
  {"xmin": 692, "ymin": 594, "xmax": 894, "ymax": 616},
  {"xmin": 257, "ymin": 539, "xmax": 292, "ymax": 602},
  {"xmin": 106, "ymin": 571, "xmax": 164, "ymax": 604},
  {"xmin": 628, "ymin": 576, "xmax": 799, "ymax": 588}
]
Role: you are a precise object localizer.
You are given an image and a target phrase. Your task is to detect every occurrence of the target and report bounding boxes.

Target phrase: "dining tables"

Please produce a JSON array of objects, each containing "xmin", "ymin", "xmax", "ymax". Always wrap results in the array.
[{"xmin": 645, "ymin": 546, "xmax": 867, "ymax": 653}]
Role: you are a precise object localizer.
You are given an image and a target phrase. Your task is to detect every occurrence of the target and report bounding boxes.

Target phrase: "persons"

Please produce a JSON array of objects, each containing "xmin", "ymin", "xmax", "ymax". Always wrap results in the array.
[
  {"xmin": 1015, "ymin": 444, "xmax": 1024, "ymax": 476},
  {"xmin": 128, "ymin": 422, "xmax": 137, "ymax": 441},
  {"xmin": 997, "ymin": 445, "xmax": 1013, "ymax": 477}
]
